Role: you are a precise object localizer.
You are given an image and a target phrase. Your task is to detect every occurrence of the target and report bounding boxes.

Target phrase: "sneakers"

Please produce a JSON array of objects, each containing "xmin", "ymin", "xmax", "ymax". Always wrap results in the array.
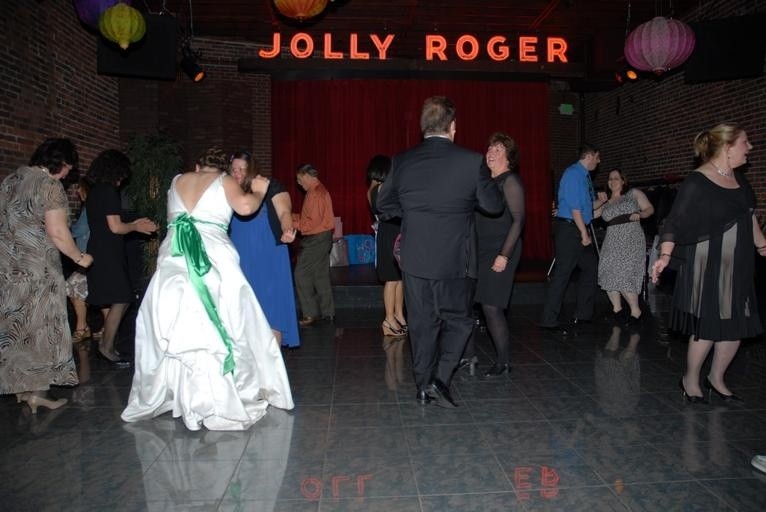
[{"xmin": 751, "ymin": 454, "xmax": 766, "ymax": 472}]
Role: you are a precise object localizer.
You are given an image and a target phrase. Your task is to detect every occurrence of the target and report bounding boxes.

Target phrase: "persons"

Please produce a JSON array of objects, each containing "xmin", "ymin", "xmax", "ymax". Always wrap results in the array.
[
  {"xmin": 537, "ymin": 140, "xmax": 653, "ymax": 332},
  {"xmin": 228, "ymin": 150, "xmax": 299, "ymax": 351},
  {"xmin": 65, "ymin": 149, "xmax": 158, "ymax": 365},
  {"xmin": 122, "ymin": 145, "xmax": 296, "ymax": 431},
  {"xmin": 366, "ymin": 97, "xmax": 527, "ymax": 408},
  {"xmin": 650, "ymin": 123, "xmax": 766, "ymax": 405},
  {"xmin": 293, "ymin": 165, "xmax": 335, "ymax": 327},
  {"xmin": 0, "ymin": 137, "xmax": 93, "ymax": 414}
]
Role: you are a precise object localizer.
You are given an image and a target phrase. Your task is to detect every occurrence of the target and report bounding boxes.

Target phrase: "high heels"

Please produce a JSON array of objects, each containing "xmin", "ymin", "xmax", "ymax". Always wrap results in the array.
[
  {"xmin": 706, "ymin": 371, "xmax": 736, "ymax": 399},
  {"xmin": 15, "ymin": 392, "xmax": 30, "ymax": 403},
  {"xmin": 381, "ymin": 316, "xmax": 408, "ymax": 337},
  {"xmin": 27, "ymin": 395, "xmax": 67, "ymax": 414},
  {"xmin": 680, "ymin": 374, "xmax": 705, "ymax": 404}
]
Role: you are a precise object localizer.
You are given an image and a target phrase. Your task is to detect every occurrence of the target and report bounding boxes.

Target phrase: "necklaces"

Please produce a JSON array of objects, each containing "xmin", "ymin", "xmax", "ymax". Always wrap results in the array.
[{"xmin": 711, "ymin": 162, "xmax": 728, "ymax": 176}]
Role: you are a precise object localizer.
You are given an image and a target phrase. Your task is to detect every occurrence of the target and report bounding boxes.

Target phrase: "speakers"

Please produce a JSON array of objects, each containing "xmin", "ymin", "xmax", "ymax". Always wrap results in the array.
[
  {"xmin": 96, "ymin": 13, "xmax": 183, "ymax": 81},
  {"xmin": 685, "ymin": 15, "xmax": 763, "ymax": 83}
]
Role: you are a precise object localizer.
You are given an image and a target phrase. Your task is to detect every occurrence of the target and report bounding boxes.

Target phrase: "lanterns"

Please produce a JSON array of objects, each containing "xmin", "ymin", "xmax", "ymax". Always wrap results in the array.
[
  {"xmin": 273, "ymin": 0, "xmax": 327, "ymax": 24},
  {"xmin": 95, "ymin": 1, "xmax": 148, "ymax": 52},
  {"xmin": 622, "ymin": 16, "xmax": 696, "ymax": 76},
  {"xmin": 74, "ymin": 1, "xmax": 116, "ymax": 33}
]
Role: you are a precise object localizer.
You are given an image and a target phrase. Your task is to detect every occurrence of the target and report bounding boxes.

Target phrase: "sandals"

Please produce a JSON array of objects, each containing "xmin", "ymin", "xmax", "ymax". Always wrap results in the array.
[{"xmin": 72, "ymin": 325, "xmax": 91, "ymax": 343}]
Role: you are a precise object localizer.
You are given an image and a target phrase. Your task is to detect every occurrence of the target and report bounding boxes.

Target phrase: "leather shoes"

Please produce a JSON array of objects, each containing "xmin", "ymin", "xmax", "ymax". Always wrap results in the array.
[
  {"xmin": 415, "ymin": 388, "xmax": 431, "ymax": 406},
  {"xmin": 484, "ymin": 361, "xmax": 512, "ymax": 378},
  {"xmin": 537, "ymin": 308, "xmax": 644, "ymax": 336},
  {"xmin": 320, "ymin": 313, "xmax": 337, "ymax": 322},
  {"xmin": 298, "ymin": 315, "xmax": 315, "ymax": 326},
  {"xmin": 96, "ymin": 346, "xmax": 130, "ymax": 368},
  {"xmin": 456, "ymin": 355, "xmax": 479, "ymax": 367},
  {"xmin": 425, "ymin": 376, "xmax": 459, "ymax": 410}
]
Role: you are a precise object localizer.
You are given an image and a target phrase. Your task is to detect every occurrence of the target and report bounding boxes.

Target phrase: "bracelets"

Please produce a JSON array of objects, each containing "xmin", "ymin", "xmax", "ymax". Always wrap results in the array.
[{"xmin": 660, "ymin": 254, "xmax": 671, "ymax": 257}]
